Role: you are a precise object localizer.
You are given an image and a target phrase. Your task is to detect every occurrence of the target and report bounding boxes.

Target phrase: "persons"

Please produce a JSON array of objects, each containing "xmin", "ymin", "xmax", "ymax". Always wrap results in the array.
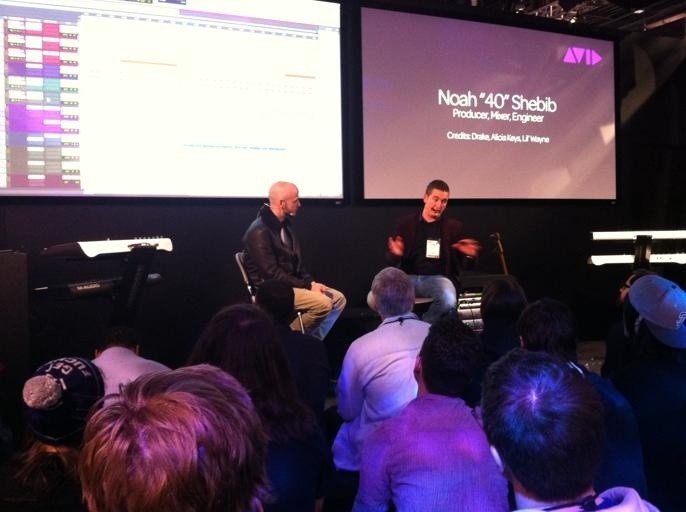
[
  {"xmin": 255, "ymin": 278, "xmax": 328, "ymax": 422},
  {"xmin": 331, "ymin": 267, "xmax": 432, "ymax": 471},
  {"xmin": 520, "ymin": 297, "xmax": 557, "ymax": 354},
  {"xmin": 482, "ymin": 349, "xmax": 658, "ymax": 512},
  {"xmin": 1, "ymin": 357, "xmax": 107, "ymax": 512},
  {"xmin": 602, "ymin": 276, "xmax": 686, "ymax": 511},
  {"xmin": 242, "ymin": 181, "xmax": 346, "ymax": 341},
  {"xmin": 89, "ymin": 318, "xmax": 174, "ymax": 410},
  {"xmin": 414, "ymin": 275, "xmax": 482, "ymax": 408},
  {"xmin": 204, "ymin": 304, "xmax": 348, "ymax": 512},
  {"xmin": 480, "ymin": 275, "xmax": 528, "ymax": 364},
  {"xmin": 80, "ymin": 364, "xmax": 270, "ymax": 512},
  {"xmin": 373, "ymin": 180, "xmax": 481, "ymax": 323},
  {"xmin": 353, "ymin": 318, "xmax": 509, "ymax": 511},
  {"xmin": 619, "ymin": 255, "xmax": 649, "ymax": 336}
]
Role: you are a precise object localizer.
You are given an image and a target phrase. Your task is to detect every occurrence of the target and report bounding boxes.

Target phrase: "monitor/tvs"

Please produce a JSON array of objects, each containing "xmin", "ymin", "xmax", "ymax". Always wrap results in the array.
[
  {"xmin": 352, "ymin": 1, "xmax": 623, "ymax": 205},
  {"xmin": 1, "ymin": 1, "xmax": 349, "ymax": 209}
]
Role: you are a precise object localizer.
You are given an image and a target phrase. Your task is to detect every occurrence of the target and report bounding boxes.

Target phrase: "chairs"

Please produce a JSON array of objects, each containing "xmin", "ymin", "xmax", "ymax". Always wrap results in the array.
[
  {"xmin": 236, "ymin": 251, "xmax": 306, "ymax": 335},
  {"xmin": 415, "ymin": 297, "xmax": 433, "ymax": 306}
]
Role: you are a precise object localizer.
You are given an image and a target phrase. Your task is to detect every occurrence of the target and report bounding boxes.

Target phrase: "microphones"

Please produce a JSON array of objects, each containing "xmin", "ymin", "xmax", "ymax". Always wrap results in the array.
[{"xmin": 490, "ymin": 230, "xmax": 503, "ymax": 252}]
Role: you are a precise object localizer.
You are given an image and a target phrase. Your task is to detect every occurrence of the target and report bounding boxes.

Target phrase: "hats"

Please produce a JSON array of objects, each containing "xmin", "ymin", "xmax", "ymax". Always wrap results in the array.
[
  {"xmin": 628, "ymin": 275, "xmax": 686, "ymax": 348},
  {"xmin": 20, "ymin": 356, "xmax": 105, "ymax": 445}
]
